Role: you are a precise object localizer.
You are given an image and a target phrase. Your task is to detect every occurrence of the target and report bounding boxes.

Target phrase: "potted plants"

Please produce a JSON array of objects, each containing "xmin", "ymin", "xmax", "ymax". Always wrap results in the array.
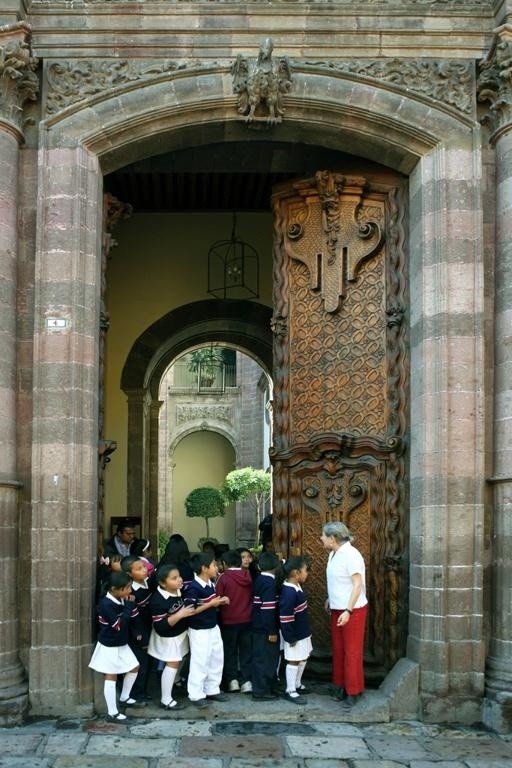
[{"xmin": 186, "ymin": 347, "xmax": 224, "ymax": 387}]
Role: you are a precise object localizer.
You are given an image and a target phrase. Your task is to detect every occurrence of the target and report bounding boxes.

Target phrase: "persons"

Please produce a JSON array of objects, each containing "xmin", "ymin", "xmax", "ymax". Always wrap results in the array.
[
  {"xmin": 182, "ymin": 547, "xmax": 232, "ymax": 709},
  {"xmin": 274, "ymin": 555, "xmax": 316, "ymax": 705},
  {"xmin": 320, "ymin": 520, "xmax": 369, "ymax": 710},
  {"xmin": 87, "ymin": 570, "xmax": 147, "ymax": 727},
  {"xmin": 96, "ymin": 515, "xmax": 285, "ymax": 701},
  {"xmin": 146, "ymin": 563, "xmax": 196, "ymax": 711}
]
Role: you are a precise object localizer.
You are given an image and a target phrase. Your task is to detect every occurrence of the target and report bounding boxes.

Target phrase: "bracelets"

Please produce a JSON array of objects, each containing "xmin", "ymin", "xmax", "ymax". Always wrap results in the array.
[{"xmin": 345, "ymin": 608, "xmax": 353, "ymax": 616}]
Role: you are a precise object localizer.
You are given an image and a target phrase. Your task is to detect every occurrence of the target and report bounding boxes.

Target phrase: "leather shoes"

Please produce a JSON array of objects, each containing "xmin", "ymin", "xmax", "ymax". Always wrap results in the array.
[
  {"xmin": 343, "ymin": 694, "xmax": 362, "ymax": 707},
  {"xmin": 332, "ymin": 688, "xmax": 344, "ymax": 701},
  {"xmin": 105, "ymin": 678, "xmax": 312, "ymax": 724}
]
